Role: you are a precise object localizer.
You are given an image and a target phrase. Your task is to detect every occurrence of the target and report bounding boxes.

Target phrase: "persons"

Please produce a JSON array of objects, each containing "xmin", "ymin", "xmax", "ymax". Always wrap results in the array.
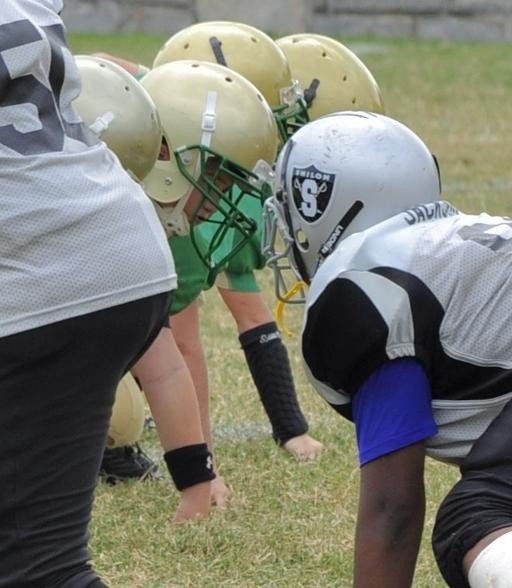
[
  {"xmin": 272, "ymin": 32, "xmax": 391, "ymax": 147},
  {"xmin": 63, "ymin": 45, "xmax": 230, "ymax": 530},
  {"xmin": 138, "ymin": 56, "xmax": 283, "ymax": 514},
  {"xmin": 93, "ymin": 17, "xmax": 332, "ymax": 486},
  {"xmin": 252, "ymin": 110, "xmax": 512, "ymax": 585},
  {"xmin": 0, "ymin": 0, "xmax": 184, "ymax": 588}
]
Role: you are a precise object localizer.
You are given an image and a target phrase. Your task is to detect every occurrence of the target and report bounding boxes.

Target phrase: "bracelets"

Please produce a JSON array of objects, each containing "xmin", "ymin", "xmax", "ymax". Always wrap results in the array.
[
  {"xmin": 159, "ymin": 440, "xmax": 221, "ymax": 492},
  {"xmin": 237, "ymin": 318, "xmax": 309, "ymax": 448}
]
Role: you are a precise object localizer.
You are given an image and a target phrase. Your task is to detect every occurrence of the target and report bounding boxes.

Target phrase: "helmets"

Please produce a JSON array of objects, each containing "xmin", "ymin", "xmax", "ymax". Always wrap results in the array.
[
  {"xmin": 151, "ymin": 20, "xmax": 297, "ymax": 111},
  {"xmin": 130, "ymin": 54, "xmax": 280, "ymax": 205},
  {"xmin": 271, "ymin": 108, "xmax": 438, "ymax": 288},
  {"xmin": 66, "ymin": 52, "xmax": 163, "ymax": 187},
  {"xmin": 274, "ymin": 32, "xmax": 384, "ymax": 134}
]
